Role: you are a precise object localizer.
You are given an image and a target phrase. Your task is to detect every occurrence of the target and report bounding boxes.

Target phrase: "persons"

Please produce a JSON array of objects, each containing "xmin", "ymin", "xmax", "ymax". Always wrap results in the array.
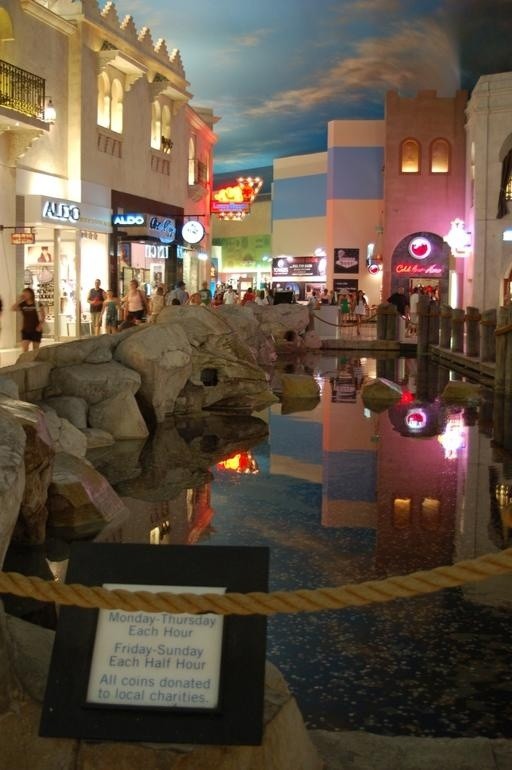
[
  {"xmin": 11, "ymin": 288, "xmax": 47, "ymax": 352},
  {"xmin": 37, "ymin": 247, "xmax": 52, "ymax": 263}
]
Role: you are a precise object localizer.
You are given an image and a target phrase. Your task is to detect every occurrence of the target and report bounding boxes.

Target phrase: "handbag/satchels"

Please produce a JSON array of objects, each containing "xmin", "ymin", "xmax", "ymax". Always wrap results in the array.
[{"xmin": 34, "ymin": 300, "xmax": 52, "ymax": 334}]
